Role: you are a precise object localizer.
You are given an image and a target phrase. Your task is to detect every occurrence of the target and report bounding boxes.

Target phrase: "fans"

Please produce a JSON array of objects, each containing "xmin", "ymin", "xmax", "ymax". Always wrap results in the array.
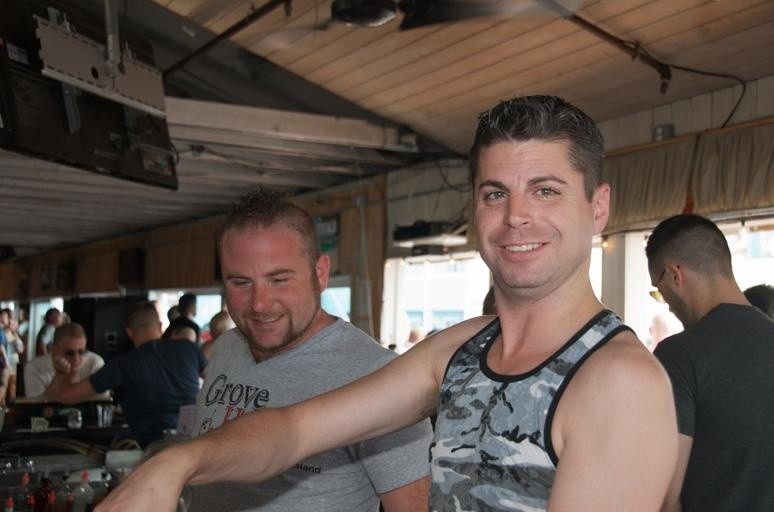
[{"xmin": 234, "ymin": 0, "xmax": 585, "ymax": 61}]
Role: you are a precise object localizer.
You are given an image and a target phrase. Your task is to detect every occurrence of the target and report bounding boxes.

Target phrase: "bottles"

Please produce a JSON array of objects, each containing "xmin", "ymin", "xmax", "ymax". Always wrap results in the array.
[
  {"xmin": 0, "ymin": 458, "xmax": 109, "ymax": 511},
  {"xmin": 67, "ymin": 410, "xmax": 83, "ymax": 429}
]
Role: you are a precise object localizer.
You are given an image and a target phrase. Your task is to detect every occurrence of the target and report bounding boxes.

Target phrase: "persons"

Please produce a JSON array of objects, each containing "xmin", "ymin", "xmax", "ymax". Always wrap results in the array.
[
  {"xmin": 99, "ymin": 96, "xmax": 678, "ymax": 511},
  {"xmin": 0, "ymin": 292, "xmax": 234, "ymax": 429},
  {"xmin": 402, "ymin": 328, "xmax": 423, "ymax": 348},
  {"xmin": 189, "ymin": 200, "xmax": 433, "ymax": 512},
  {"xmin": 645, "ymin": 214, "xmax": 774, "ymax": 512}
]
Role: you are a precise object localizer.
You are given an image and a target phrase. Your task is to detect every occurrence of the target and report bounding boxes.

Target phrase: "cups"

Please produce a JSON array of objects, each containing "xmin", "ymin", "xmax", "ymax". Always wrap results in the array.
[{"xmin": 97, "ymin": 404, "xmax": 116, "ymax": 428}]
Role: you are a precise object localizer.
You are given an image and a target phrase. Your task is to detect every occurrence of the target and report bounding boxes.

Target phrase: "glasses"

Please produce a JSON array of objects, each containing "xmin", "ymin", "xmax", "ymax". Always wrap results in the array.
[
  {"xmin": 64, "ymin": 348, "xmax": 85, "ymax": 357},
  {"xmin": 649, "ymin": 265, "xmax": 680, "ymax": 304}
]
